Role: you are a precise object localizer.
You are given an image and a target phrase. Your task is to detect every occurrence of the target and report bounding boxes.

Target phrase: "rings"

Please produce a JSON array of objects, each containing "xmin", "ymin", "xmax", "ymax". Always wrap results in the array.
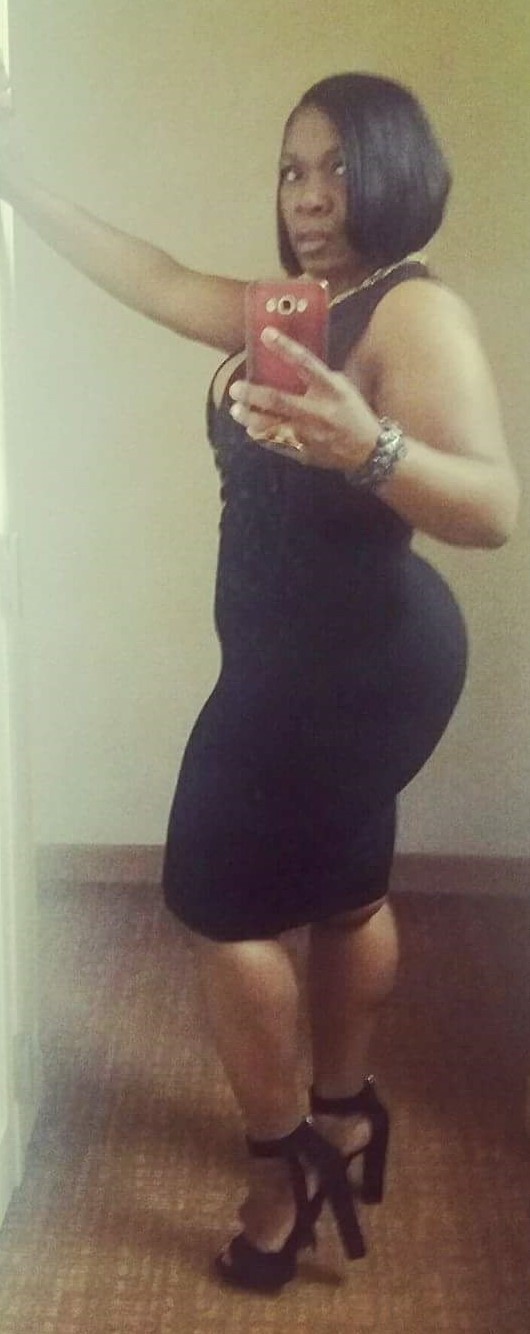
[{"xmin": 248, "ymin": 414, "xmax": 303, "ymax": 455}]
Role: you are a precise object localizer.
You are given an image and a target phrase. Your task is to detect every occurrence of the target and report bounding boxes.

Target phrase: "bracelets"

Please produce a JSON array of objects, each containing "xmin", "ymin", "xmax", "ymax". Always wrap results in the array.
[{"xmin": 348, "ymin": 418, "xmax": 406, "ymax": 497}]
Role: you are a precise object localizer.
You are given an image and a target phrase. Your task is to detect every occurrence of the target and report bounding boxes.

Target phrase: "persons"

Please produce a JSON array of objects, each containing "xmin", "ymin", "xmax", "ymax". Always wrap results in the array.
[{"xmin": 0, "ymin": 70, "xmax": 520, "ymax": 1288}]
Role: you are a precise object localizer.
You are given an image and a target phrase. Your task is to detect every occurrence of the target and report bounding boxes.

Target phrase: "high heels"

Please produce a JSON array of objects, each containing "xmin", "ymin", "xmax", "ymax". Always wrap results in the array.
[
  {"xmin": 306, "ymin": 1073, "xmax": 387, "ymax": 1208},
  {"xmin": 215, "ymin": 1117, "xmax": 366, "ymax": 1296}
]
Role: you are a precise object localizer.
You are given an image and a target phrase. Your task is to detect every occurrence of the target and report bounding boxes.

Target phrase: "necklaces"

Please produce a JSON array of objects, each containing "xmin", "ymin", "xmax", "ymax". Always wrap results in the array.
[{"xmin": 324, "ymin": 256, "xmax": 425, "ymax": 308}]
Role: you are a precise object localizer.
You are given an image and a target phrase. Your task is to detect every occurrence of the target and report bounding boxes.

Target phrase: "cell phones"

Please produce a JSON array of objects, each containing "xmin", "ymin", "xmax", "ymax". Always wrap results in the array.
[{"xmin": 244, "ymin": 280, "xmax": 329, "ymax": 419}]
